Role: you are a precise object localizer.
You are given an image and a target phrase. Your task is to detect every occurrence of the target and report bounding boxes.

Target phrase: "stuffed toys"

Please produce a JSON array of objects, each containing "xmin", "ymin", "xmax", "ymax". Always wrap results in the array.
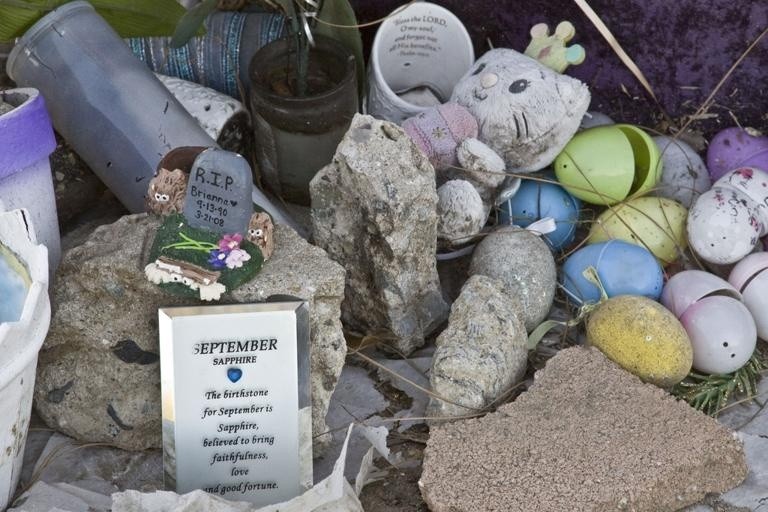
[{"xmin": 400, "ymin": 47, "xmax": 591, "ymax": 244}]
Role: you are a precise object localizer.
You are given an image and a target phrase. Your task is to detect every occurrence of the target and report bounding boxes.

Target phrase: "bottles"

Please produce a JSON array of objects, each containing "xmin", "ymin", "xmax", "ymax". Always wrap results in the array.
[
  {"xmin": 5, "ymin": 1, "xmax": 293, "ymax": 233},
  {"xmin": 248, "ymin": 34, "xmax": 359, "ymax": 204}
]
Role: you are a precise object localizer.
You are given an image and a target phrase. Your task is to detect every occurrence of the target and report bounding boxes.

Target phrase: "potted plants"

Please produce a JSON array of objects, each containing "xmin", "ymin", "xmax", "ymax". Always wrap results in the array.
[{"xmin": 163, "ymin": 0, "xmax": 362, "ymax": 207}]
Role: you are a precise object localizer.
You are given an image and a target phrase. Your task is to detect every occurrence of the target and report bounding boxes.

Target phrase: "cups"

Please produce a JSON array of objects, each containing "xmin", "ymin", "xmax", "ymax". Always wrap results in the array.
[{"xmin": 363, "ymin": 1, "xmax": 477, "ymax": 127}]
